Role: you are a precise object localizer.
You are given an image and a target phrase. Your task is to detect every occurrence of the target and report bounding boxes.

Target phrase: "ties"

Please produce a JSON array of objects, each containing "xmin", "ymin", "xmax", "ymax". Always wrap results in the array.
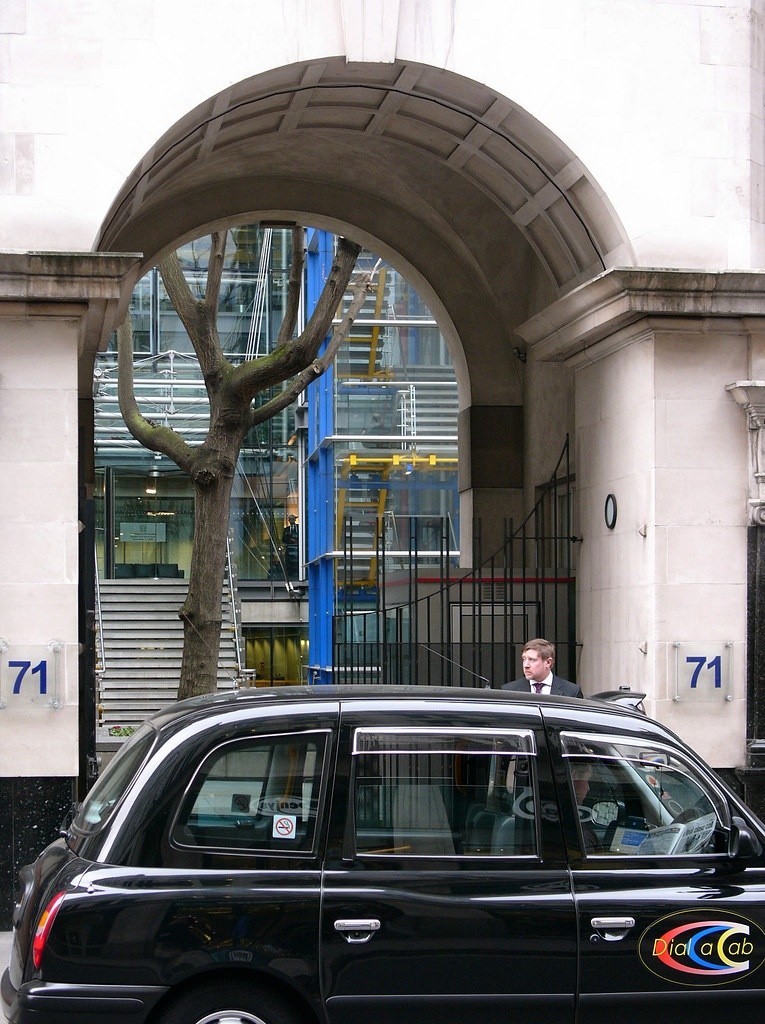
[{"xmin": 534, "ymin": 683, "xmax": 544, "ymax": 693}]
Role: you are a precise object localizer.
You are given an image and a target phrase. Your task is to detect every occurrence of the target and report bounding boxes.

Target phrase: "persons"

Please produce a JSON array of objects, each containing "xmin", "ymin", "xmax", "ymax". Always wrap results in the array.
[
  {"xmin": 282, "ymin": 514, "xmax": 299, "ymax": 579},
  {"xmin": 501, "ymin": 639, "xmax": 583, "ymax": 698},
  {"xmin": 360, "ymin": 414, "xmax": 391, "ymax": 509},
  {"xmin": 568, "ymin": 750, "xmax": 611, "ymax": 856}
]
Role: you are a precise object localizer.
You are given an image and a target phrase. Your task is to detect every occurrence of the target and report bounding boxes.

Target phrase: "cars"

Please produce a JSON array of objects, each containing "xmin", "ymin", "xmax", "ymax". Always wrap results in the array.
[{"xmin": 0, "ymin": 685, "xmax": 765, "ymax": 1024}]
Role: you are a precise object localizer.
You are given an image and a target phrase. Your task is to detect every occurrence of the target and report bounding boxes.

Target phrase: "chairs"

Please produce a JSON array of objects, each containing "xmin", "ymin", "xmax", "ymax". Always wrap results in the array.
[
  {"xmin": 463, "ymin": 803, "xmax": 524, "ymax": 857},
  {"xmin": 115, "ymin": 563, "xmax": 185, "ymax": 578}
]
